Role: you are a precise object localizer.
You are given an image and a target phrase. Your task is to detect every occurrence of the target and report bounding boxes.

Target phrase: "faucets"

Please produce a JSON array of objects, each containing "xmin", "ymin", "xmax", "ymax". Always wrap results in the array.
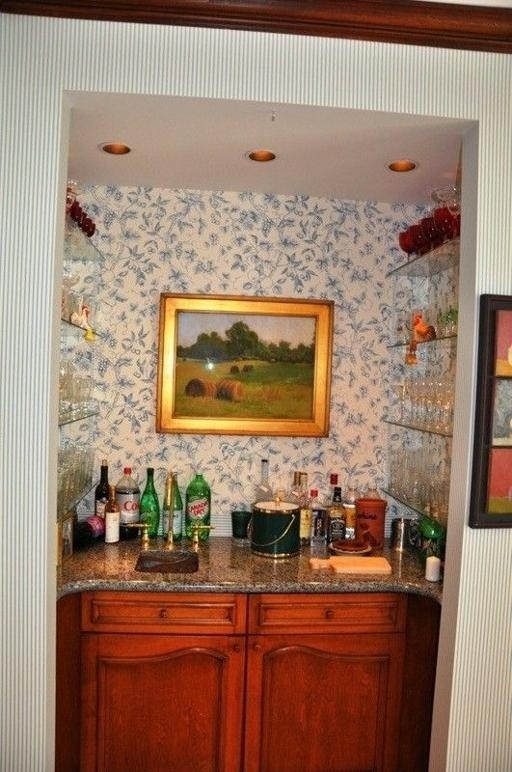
[{"xmin": 162, "ymin": 472, "xmax": 177, "ymax": 552}]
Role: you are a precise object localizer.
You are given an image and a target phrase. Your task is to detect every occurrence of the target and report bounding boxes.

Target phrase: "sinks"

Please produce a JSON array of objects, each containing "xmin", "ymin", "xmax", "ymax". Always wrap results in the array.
[{"xmin": 133, "ymin": 548, "xmax": 200, "ymax": 575}]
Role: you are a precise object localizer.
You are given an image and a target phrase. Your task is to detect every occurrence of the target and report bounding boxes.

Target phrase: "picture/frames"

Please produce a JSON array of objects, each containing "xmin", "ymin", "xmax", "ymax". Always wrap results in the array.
[
  {"xmin": 155, "ymin": 291, "xmax": 335, "ymax": 437},
  {"xmin": 469, "ymin": 296, "xmax": 512, "ymax": 530}
]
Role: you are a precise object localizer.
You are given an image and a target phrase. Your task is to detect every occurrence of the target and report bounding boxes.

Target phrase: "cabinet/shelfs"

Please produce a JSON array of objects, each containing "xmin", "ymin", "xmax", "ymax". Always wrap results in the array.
[
  {"xmin": 380, "ymin": 241, "xmax": 459, "ymax": 531},
  {"xmin": 79, "ymin": 593, "xmax": 410, "ymax": 771},
  {"xmin": 57, "ymin": 192, "xmax": 103, "ymax": 517}
]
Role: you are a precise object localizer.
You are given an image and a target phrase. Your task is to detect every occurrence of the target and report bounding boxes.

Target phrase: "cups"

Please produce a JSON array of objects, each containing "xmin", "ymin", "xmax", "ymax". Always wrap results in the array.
[
  {"xmin": 55, "ymin": 193, "xmax": 99, "ymax": 512},
  {"xmin": 390, "ymin": 518, "xmax": 411, "ymax": 553},
  {"xmin": 232, "ymin": 511, "xmax": 253, "ymax": 547},
  {"xmin": 389, "ymin": 459, "xmax": 449, "ymax": 524},
  {"xmin": 355, "ymin": 500, "xmax": 387, "ymax": 546}
]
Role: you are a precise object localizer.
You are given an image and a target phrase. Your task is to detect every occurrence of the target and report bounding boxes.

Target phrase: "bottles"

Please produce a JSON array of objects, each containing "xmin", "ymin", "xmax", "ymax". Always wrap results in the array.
[
  {"xmin": 73, "ymin": 458, "xmax": 211, "ymax": 549},
  {"xmin": 255, "ymin": 458, "xmax": 382, "ymax": 543}
]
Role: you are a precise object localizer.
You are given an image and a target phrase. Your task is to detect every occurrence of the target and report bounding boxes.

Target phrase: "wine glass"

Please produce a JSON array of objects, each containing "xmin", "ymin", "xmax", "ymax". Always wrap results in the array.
[
  {"xmin": 397, "ymin": 209, "xmax": 462, "ymax": 264},
  {"xmin": 395, "ymin": 377, "xmax": 455, "ymax": 434}
]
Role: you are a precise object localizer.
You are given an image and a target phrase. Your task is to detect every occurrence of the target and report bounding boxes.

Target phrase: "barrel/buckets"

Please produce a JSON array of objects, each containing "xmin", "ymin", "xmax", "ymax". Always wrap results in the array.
[{"xmin": 246, "ymin": 500, "xmax": 301, "ymax": 559}]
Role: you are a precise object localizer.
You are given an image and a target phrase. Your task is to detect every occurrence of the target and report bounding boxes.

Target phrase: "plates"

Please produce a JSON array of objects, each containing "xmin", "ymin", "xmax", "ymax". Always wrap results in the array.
[{"xmin": 328, "ymin": 542, "xmax": 372, "ymax": 556}]
